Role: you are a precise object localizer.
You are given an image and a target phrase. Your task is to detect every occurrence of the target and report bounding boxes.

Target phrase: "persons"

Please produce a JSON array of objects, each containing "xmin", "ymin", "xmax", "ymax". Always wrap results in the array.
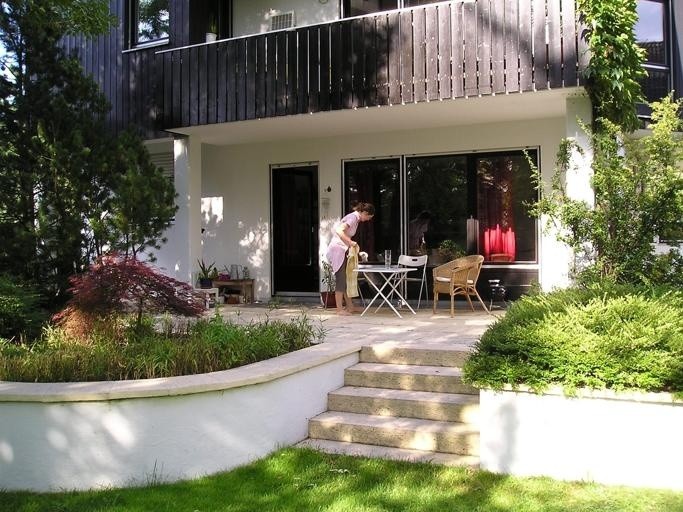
[
  {"xmin": 325, "ymin": 200, "xmax": 374, "ymax": 315},
  {"xmin": 408, "ymin": 209, "xmax": 431, "ymax": 256}
]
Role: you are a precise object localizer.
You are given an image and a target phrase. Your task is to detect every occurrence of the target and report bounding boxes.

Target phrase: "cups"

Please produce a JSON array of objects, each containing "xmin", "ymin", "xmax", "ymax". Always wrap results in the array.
[{"xmin": 385, "ymin": 250, "xmax": 391, "ymax": 270}]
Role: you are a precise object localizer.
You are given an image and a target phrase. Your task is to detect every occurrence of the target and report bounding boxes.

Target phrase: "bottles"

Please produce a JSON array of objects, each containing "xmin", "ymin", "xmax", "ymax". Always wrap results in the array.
[{"xmin": 243, "ymin": 267, "xmax": 249, "ymax": 279}]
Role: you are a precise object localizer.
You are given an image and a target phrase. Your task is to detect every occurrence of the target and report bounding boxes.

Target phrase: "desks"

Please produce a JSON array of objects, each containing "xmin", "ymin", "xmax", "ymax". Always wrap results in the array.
[{"xmin": 211, "ymin": 278, "xmax": 255, "ymax": 305}]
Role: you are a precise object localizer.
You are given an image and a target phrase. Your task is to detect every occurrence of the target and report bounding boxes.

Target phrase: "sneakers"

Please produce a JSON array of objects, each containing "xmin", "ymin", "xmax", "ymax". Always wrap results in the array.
[{"xmin": 336, "ymin": 306, "xmax": 365, "ymax": 316}]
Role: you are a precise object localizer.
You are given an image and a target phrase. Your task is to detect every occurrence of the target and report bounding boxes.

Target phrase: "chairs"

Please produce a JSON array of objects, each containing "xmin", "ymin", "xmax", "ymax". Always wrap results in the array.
[
  {"xmin": 391, "ymin": 255, "xmax": 428, "ymax": 309},
  {"xmin": 433, "ymin": 255, "xmax": 489, "ymax": 318}
]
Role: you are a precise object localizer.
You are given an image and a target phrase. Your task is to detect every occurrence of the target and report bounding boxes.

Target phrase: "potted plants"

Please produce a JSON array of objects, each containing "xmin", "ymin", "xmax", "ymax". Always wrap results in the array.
[
  {"xmin": 195, "ymin": 255, "xmax": 216, "ymax": 288},
  {"xmin": 205, "ymin": 25, "xmax": 218, "ymax": 42},
  {"xmin": 319, "ymin": 259, "xmax": 338, "ymax": 308}
]
